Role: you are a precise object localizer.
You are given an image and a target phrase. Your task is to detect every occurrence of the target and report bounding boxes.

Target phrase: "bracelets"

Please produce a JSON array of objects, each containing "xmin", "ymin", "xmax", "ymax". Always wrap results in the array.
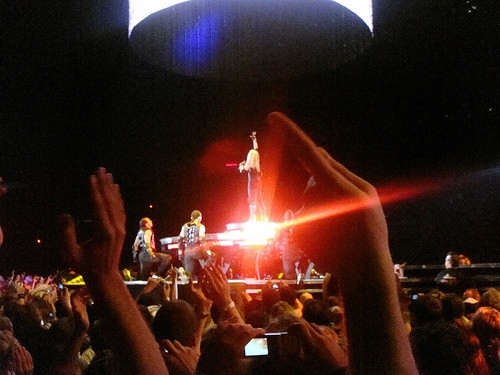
[{"xmin": 212, "ymin": 301, "xmax": 236, "ymax": 313}]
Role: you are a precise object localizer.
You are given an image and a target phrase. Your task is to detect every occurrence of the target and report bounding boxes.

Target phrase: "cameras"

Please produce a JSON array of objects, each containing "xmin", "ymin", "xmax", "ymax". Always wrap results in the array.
[{"xmin": 242, "ymin": 332, "xmax": 303, "ymax": 357}]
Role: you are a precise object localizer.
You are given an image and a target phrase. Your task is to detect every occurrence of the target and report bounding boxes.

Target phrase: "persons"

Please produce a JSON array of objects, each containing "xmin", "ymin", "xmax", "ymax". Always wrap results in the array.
[
  {"xmin": 238, "ymin": 128, "xmax": 269, "ymax": 223},
  {"xmin": 0, "ymin": 112, "xmax": 500, "ymax": 375}
]
